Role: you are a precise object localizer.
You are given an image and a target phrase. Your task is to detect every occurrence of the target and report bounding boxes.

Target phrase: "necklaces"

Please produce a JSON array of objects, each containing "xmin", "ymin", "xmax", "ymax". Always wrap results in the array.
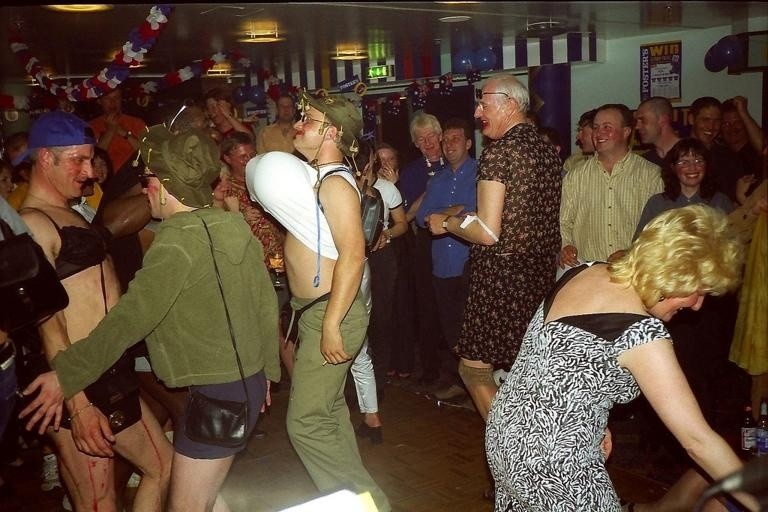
[{"xmin": 312, "ymin": 159, "xmax": 344, "ymax": 168}]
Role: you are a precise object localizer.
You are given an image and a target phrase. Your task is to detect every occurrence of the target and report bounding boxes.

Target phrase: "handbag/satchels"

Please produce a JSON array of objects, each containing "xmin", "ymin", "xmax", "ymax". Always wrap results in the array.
[{"xmin": 184, "ymin": 390, "xmax": 251, "ymax": 448}]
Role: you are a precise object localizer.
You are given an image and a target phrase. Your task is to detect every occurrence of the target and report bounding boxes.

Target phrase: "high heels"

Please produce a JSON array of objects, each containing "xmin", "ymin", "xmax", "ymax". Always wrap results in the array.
[{"xmin": 356, "ymin": 420, "xmax": 385, "ymax": 444}]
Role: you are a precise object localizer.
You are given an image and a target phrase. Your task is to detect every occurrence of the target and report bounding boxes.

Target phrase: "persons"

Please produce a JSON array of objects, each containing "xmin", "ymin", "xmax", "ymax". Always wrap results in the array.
[
  {"xmin": 245, "ymin": 88, "xmax": 395, "ymax": 512},
  {"xmin": 8, "ymin": 113, "xmax": 175, "ymax": 509},
  {"xmin": 20, "ymin": 125, "xmax": 281, "ymax": 511},
  {"xmin": 1, "ymin": 82, "xmax": 767, "ymax": 512},
  {"xmin": 425, "ymin": 76, "xmax": 560, "ymax": 433},
  {"xmin": 474, "ymin": 203, "xmax": 765, "ymax": 510}
]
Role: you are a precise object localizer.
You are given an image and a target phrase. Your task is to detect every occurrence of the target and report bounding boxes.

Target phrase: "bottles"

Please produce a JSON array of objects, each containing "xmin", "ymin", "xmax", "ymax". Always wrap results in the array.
[
  {"xmin": 738, "ymin": 405, "xmax": 757, "ymax": 450},
  {"xmin": 757, "ymin": 401, "xmax": 768, "ymax": 453}
]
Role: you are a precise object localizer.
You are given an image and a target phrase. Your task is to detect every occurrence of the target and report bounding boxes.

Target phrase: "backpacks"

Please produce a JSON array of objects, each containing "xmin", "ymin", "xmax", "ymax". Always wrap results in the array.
[
  {"xmin": 0, "ymin": 216, "xmax": 70, "ymax": 340},
  {"xmin": 318, "ymin": 168, "xmax": 384, "ymax": 258}
]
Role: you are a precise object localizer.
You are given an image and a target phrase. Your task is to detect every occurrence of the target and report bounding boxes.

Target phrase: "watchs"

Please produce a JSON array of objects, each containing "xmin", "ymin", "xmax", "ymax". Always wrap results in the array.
[{"xmin": 442, "ymin": 213, "xmax": 453, "ymax": 239}]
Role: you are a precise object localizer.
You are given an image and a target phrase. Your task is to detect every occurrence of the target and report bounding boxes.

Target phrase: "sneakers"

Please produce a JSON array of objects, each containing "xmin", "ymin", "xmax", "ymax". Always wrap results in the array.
[
  {"xmin": 491, "ymin": 369, "xmax": 510, "ymax": 388},
  {"xmin": 41, "ymin": 454, "xmax": 66, "ymax": 494},
  {"xmin": 431, "ymin": 384, "xmax": 470, "ymax": 400},
  {"xmin": 254, "ymin": 395, "xmax": 272, "ymax": 438}
]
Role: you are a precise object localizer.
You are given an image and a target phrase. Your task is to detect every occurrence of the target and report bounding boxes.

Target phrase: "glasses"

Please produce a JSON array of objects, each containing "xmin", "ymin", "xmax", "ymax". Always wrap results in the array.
[
  {"xmin": 675, "ymin": 157, "xmax": 705, "ymax": 167},
  {"xmin": 137, "ymin": 172, "xmax": 156, "ymax": 189},
  {"xmin": 475, "ymin": 88, "xmax": 510, "ymax": 100}
]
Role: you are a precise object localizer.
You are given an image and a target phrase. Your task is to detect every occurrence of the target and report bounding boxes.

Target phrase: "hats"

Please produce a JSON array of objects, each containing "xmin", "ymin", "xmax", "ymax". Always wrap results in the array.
[
  {"xmin": 137, "ymin": 123, "xmax": 223, "ymax": 209},
  {"xmin": 298, "ymin": 91, "xmax": 364, "ymax": 158},
  {"xmin": 11, "ymin": 110, "xmax": 93, "ymax": 164}
]
